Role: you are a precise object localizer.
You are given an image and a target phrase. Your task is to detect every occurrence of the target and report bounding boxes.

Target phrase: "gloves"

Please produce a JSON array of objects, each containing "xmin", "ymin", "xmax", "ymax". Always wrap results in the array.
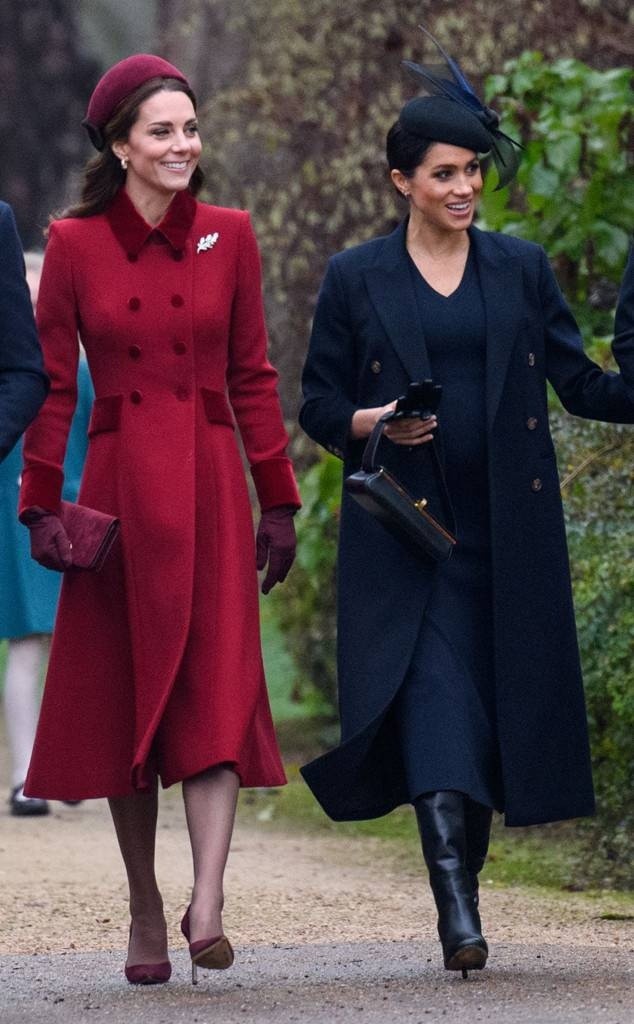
[
  {"xmin": 380, "ymin": 379, "xmax": 444, "ymax": 455},
  {"xmin": 256, "ymin": 503, "xmax": 297, "ymax": 596},
  {"xmin": 30, "ymin": 514, "xmax": 73, "ymax": 572}
]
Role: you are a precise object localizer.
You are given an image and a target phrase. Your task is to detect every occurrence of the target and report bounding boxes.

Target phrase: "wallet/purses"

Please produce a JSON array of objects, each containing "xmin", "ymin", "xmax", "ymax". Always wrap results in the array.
[{"xmin": 58, "ymin": 499, "xmax": 120, "ymax": 573}]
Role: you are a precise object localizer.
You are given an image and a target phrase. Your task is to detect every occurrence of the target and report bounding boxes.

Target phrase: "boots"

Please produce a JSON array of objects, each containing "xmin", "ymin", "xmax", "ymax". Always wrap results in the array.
[{"xmin": 415, "ymin": 790, "xmax": 494, "ymax": 978}]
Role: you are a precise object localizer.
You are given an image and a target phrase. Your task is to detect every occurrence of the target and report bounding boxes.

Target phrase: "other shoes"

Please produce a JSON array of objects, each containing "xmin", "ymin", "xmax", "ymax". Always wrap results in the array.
[{"xmin": 9, "ymin": 784, "xmax": 49, "ymax": 814}]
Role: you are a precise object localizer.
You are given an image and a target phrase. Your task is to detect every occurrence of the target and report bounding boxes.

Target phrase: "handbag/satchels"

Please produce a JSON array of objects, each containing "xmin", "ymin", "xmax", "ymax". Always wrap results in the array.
[{"xmin": 344, "ymin": 409, "xmax": 459, "ymax": 577}]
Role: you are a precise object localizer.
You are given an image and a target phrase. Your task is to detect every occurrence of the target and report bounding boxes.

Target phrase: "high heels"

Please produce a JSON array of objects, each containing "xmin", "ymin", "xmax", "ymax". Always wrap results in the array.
[
  {"xmin": 124, "ymin": 916, "xmax": 172, "ymax": 984},
  {"xmin": 180, "ymin": 902, "xmax": 235, "ymax": 985}
]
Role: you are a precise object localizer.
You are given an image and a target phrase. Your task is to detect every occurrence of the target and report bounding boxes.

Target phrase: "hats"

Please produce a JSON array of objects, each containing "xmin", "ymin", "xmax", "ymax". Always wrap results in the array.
[
  {"xmin": 81, "ymin": 53, "xmax": 190, "ymax": 152},
  {"xmin": 400, "ymin": 24, "xmax": 529, "ymax": 191}
]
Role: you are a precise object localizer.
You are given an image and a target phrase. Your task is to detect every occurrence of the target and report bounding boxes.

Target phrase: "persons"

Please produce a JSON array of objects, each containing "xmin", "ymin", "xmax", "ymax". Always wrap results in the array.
[
  {"xmin": 296, "ymin": 25, "xmax": 634, "ymax": 976},
  {"xmin": 1, "ymin": 196, "xmax": 94, "ymax": 823},
  {"xmin": 16, "ymin": 51, "xmax": 301, "ymax": 984}
]
